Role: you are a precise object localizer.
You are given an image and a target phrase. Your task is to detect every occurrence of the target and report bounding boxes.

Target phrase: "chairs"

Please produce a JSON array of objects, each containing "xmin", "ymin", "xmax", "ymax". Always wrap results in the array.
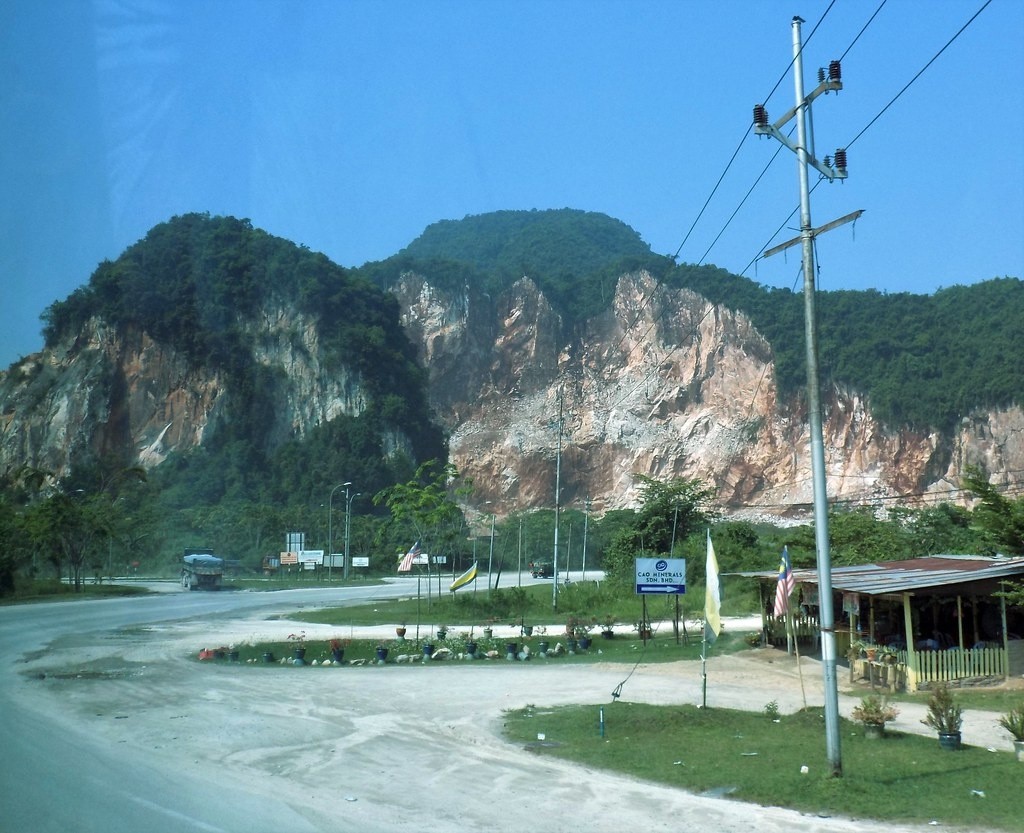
[{"xmin": 918, "ymin": 641, "xmax": 985, "ymax": 673}]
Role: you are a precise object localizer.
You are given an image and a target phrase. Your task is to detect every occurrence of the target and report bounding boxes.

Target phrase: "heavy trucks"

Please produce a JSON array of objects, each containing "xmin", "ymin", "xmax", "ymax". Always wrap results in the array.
[
  {"xmin": 178, "ymin": 546, "xmax": 224, "ymax": 592},
  {"xmin": 224, "ymin": 555, "xmax": 281, "ymax": 576}
]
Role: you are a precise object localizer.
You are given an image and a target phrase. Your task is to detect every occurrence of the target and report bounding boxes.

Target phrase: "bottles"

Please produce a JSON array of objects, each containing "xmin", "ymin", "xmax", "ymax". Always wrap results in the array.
[{"xmin": 857, "ymin": 625, "xmax": 862, "ymax": 631}]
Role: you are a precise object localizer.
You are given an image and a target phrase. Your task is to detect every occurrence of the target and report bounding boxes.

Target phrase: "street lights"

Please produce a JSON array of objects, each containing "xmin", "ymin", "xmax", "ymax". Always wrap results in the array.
[
  {"xmin": 110, "ymin": 498, "xmax": 124, "ymax": 577},
  {"xmin": 328, "ymin": 482, "xmax": 362, "ymax": 583},
  {"xmin": 33, "ymin": 488, "xmax": 84, "ymax": 567}
]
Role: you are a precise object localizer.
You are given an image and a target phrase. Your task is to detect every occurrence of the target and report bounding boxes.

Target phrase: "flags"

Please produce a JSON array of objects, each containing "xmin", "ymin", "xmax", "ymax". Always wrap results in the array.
[
  {"xmin": 397, "ymin": 540, "xmax": 421, "ymax": 571},
  {"xmin": 773, "ymin": 549, "xmax": 796, "ymax": 617},
  {"xmin": 704, "ymin": 536, "xmax": 724, "ymax": 646},
  {"xmin": 449, "ymin": 562, "xmax": 478, "ymax": 593}
]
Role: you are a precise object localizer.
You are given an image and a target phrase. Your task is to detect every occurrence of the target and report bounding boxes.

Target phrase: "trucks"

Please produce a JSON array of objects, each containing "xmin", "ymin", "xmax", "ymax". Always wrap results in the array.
[{"xmin": 530, "ymin": 562, "xmax": 559, "ymax": 579}]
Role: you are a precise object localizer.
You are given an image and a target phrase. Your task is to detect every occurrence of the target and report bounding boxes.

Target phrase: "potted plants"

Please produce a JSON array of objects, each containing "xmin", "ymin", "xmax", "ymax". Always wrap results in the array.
[
  {"xmin": 919, "ymin": 682, "xmax": 963, "ymax": 752},
  {"xmin": 851, "ymin": 695, "xmax": 902, "ymax": 740},
  {"xmin": 998, "ymin": 703, "xmax": 1024, "ymax": 765},
  {"xmin": 634, "ymin": 618, "xmax": 654, "ymax": 640},
  {"xmin": 264, "ymin": 607, "xmax": 616, "ymax": 663},
  {"xmin": 215, "ymin": 646, "xmax": 238, "ymax": 661},
  {"xmin": 845, "ymin": 639, "xmax": 899, "ymax": 664}
]
